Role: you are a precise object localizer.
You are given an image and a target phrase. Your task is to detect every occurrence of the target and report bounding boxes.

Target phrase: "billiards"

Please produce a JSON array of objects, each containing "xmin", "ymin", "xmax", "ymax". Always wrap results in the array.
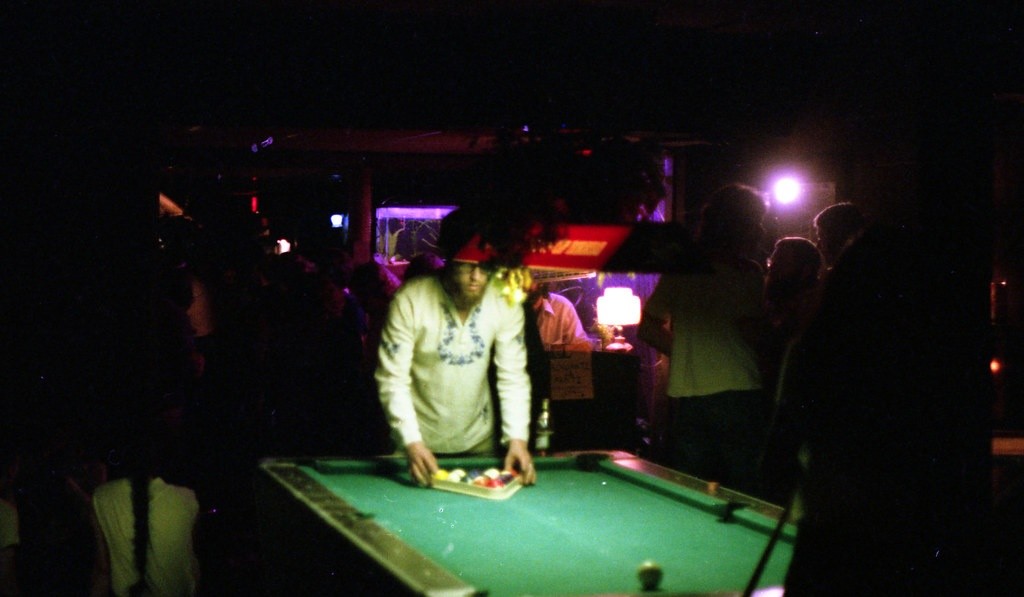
[
  {"xmin": 433, "ymin": 466, "xmax": 517, "ymax": 490},
  {"xmin": 637, "ymin": 560, "xmax": 664, "ymax": 588}
]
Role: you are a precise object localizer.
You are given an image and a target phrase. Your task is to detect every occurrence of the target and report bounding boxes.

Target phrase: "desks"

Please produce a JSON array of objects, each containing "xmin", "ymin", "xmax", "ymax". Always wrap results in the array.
[{"xmin": 255, "ymin": 450, "xmax": 803, "ymax": 597}]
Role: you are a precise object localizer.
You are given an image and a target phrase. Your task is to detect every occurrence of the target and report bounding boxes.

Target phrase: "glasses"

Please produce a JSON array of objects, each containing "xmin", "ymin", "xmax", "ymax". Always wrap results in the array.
[
  {"xmin": 446, "ymin": 260, "xmax": 495, "ymax": 275},
  {"xmin": 766, "ymin": 257, "xmax": 802, "ymax": 270}
]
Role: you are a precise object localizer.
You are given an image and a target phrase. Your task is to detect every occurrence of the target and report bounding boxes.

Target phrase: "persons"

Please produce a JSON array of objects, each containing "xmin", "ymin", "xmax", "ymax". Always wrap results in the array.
[
  {"xmin": 635, "ymin": 131, "xmax": 994, "ymax": 597},
  {"xmin": 0, "ymin": 205, "xmax": 589, "ymax": 597}
]
